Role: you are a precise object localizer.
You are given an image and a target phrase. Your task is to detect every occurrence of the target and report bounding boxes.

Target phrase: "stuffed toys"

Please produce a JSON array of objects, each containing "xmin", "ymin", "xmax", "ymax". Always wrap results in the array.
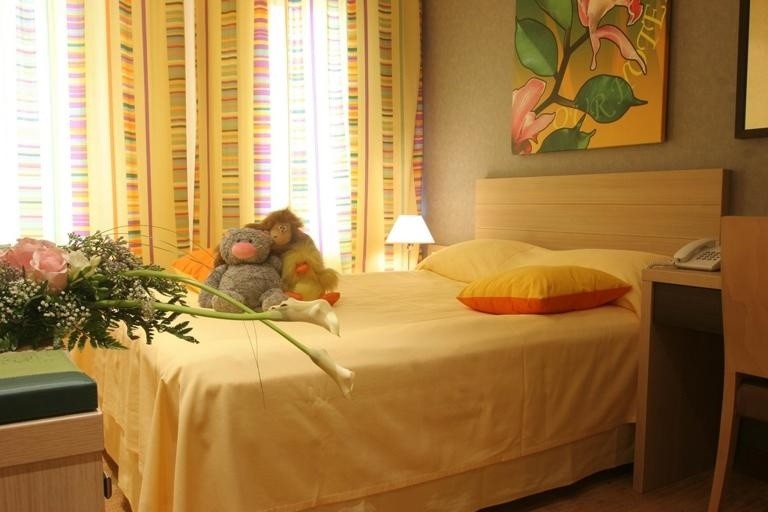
[
  {"xmin": 195, "ymin": 227, "xmax": 286, "ymax": 314},
  {"xmin": 279, "ymin": 243, "xmax": 341, "ymax": 306},
  {"xmin": 212, "ymin": 205, "xmax": 315, "ymax": 267}
]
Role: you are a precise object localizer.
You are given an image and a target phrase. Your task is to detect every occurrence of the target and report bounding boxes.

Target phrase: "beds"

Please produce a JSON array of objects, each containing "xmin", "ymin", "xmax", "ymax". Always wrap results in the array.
[{"xmin": 65, "ymin": 167, "xmax": 734, "ymax": 512}]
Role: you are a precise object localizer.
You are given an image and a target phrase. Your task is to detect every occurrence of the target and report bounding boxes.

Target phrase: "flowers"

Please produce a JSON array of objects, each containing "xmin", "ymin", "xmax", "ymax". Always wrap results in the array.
[{"xmin": 0, "ymin": 223, "xmax": 358, "ymax": 409}]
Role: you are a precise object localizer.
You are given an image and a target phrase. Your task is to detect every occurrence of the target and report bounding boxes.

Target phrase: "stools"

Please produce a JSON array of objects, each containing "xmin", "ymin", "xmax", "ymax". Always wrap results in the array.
[{"xmin": 0, "ymin": 349, "xmax": 112, "ymax": 512}]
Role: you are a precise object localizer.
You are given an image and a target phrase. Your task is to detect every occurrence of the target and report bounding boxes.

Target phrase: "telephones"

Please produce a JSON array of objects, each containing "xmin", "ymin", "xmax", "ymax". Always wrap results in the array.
[{"xmin": 673, "ymin": 238, "xmax": 720, "ymax": 271}]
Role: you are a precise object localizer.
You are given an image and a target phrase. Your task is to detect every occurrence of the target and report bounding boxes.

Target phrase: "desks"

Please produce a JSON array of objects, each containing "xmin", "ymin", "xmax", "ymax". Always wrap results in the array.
[{"xmin": 632, "ymin": 266, "xmax": 721, "ymax": 493}]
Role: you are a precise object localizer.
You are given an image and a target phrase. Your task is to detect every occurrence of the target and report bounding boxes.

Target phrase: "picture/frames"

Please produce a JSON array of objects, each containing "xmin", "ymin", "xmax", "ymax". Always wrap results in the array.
[{"xmin": 734, "ymin": 0, "xmax": 768, "ymax": 138}]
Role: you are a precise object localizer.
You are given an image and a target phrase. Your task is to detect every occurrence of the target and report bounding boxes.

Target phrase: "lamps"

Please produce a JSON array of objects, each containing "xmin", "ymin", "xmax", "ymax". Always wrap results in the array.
[{"xmin": 385, "ymin": 215, "xmax": 435, "ymax": 270}]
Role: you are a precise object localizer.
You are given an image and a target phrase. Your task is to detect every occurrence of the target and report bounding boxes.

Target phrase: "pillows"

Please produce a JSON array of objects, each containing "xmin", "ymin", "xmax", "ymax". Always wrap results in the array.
[{"xmin": 414, "ymin": 237, "xmax": 672, "ymax": 321}]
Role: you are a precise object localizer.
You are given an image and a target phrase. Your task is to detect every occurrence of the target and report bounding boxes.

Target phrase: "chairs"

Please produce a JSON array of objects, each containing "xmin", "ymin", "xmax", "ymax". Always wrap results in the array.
[{"xmin": 706, "ymin": 216, "xmax": 768, "ymax": 512}]
